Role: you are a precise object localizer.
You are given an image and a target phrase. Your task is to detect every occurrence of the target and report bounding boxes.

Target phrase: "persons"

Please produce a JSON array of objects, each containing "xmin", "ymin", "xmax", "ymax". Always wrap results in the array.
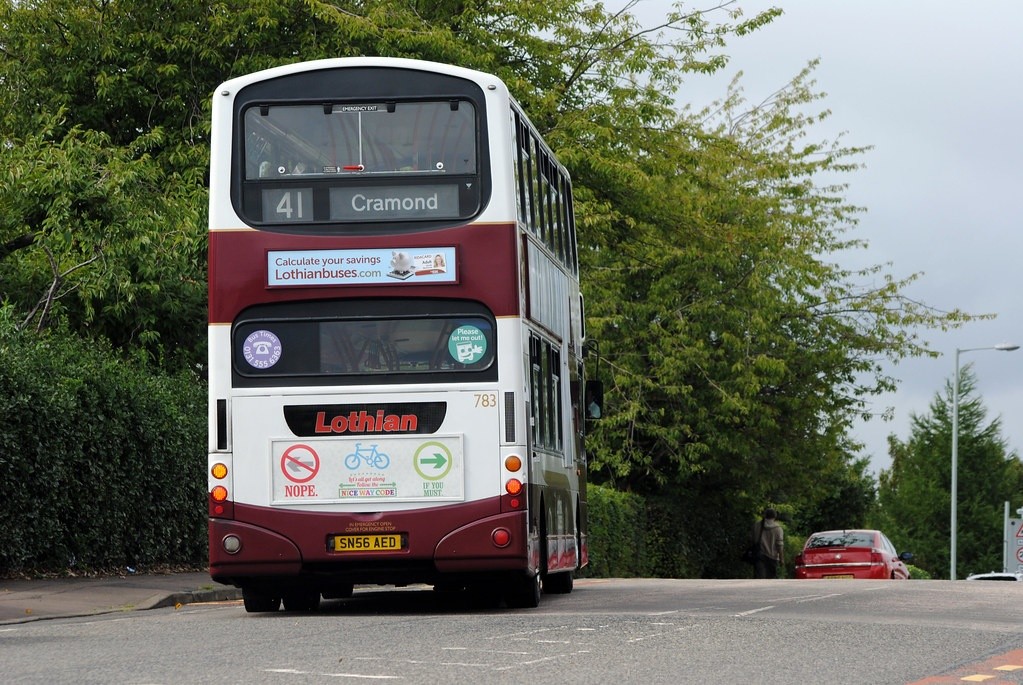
[
  {"xmin": 750, "ymin": 507, "xmax": 785, "ymax": 579},
  {"xmin": 584, "ymin": 389, "xmax": 601, "ymax": 419},
  {"xmin": 434, "ymin": 254, "xmax": 444, "ymax": 268}
]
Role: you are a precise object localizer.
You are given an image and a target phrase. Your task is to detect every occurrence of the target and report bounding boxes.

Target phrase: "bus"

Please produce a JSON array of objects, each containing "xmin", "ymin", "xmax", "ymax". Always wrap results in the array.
[{"xmin": 207, "ymin": 57, "xmax": 603, "ymax": 614}]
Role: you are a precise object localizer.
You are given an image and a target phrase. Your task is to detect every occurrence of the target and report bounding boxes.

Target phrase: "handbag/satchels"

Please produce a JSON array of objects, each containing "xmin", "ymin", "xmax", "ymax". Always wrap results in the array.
[{"xmin": 741, "ymin": 544, "xmax": 760, "ymax": 563}]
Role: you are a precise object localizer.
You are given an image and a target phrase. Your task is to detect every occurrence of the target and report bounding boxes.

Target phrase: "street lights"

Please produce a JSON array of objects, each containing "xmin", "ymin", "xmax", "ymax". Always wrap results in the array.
[{"xmin": 950, "ymin": 344, "xmax": 1021, "ymax": 580}]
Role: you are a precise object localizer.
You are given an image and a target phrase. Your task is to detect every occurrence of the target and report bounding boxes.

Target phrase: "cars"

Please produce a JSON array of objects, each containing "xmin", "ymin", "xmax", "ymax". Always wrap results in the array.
[
  {"xmin": 794, "ymin": 529, "xmax": 913, "ymax": 580},
  {"xmin": 965, "ymin": 571, "xmax": 1023, "ymax": 582}
]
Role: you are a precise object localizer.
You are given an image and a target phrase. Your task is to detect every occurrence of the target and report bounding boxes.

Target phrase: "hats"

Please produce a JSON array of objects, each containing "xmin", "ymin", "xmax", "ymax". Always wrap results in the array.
[{"xmin": 766, "ymin": 509, "xmax": 777, "ymax": 518}]
